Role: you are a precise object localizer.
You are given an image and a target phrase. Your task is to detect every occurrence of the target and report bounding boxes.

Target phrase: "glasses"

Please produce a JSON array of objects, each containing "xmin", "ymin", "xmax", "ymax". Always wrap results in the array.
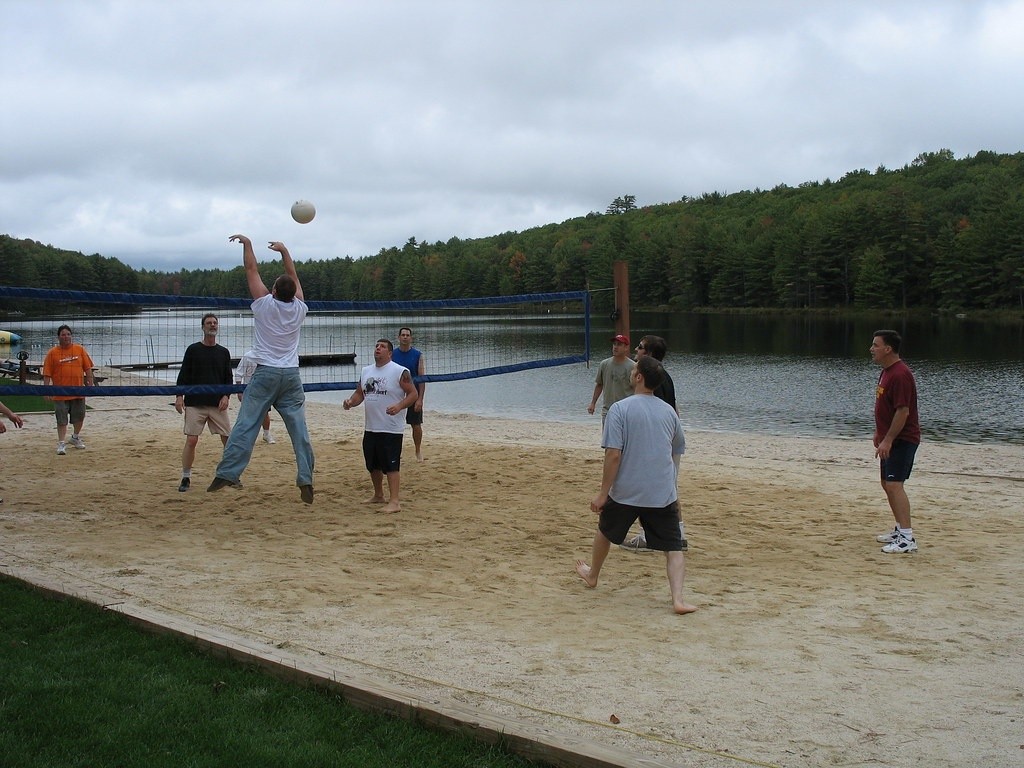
[{"xmin": 638, "ymin": 344, "xmax": 645, "ymax": 350}]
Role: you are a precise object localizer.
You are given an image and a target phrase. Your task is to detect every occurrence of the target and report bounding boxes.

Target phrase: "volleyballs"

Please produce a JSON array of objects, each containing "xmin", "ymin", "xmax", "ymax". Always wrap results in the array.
[{"xmin": 291, "ymin": 198, "xmax": 317, "ymax": 225}]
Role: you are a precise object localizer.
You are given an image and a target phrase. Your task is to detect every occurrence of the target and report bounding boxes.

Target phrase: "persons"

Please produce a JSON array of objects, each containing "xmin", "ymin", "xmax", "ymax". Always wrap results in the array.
[
  {"xmin": 391, "ymin": 328, "xmax": 425, "ymax": 462},
  {"xmin": 575, "ymin": 356, "xmax": 699, "ymax": 615},
  {"xmin": 207, "ymin": 234, "xmax": 315, "ymax": 505},
  {"xmin": 871, "ymin": 330, "xmax": 922, "ymax": 554},
  {"xmin": 175, "ymin": 314, "xmax": 244, "ymax": 492},
  {"xmin": 0, "ymin": 401, "xmax": 23, "ymax": 503},
  {"xmin": 235, "ymin": 351, "xmax": 275, "ymax": 444},
  {"xmin": 620, "ymin": 336, "xmax": 687, "ymax": 550},
  {"xmin": 587, "ymin": 336, "xmax": 636, "ymax": 488},
  {"xmin": 43, "ymin": 324, "xmax": 93, "ymax": 455},
  {"xmin": 343, "ymin": 339, "xmax": 418, "ymax": 513}
]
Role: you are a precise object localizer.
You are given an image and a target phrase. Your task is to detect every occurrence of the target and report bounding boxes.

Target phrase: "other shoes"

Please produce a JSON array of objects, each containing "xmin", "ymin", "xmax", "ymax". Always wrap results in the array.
[
  {"xmin": 207, "ymin": 477, "xmax": 235, "ymax": 492},
  {"xmin": 68, "ymin": 435, "xmax": 86, "ymax": 449},
  {"xmin": 178, "ymin": 478, "xmax": 190, "ymax": 492},
  {"xmin": 300, "ymin": 484, "xmax": 314, "ymax": 505},
  {"xmin": 263, "ymin": 433, "xmax": 276, "ymax": 444},
  {"xmin": 56, "ymin": 441, "xmax": 68, "ymax": 455}
]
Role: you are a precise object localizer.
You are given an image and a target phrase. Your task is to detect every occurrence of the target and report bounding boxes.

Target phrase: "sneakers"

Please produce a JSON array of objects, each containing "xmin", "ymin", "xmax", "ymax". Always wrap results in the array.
[
  {"xmin": 619, "ymin": 536, "xmax": 654, "ymax": 554},
  {"xmin": 880, "ymin": 534, "xmax": 918, "ymax": 553},
  {"xmin": 681, "ymin": 539, "xmax": 688, "ymax": 551},
  {"xmin": 876, "ymin": 526, "xmax": 900, "ymax": 542}
]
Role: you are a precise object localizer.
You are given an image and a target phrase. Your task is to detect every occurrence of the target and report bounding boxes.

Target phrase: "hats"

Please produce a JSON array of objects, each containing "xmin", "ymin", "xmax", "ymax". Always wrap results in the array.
[{"xmin": 611, "ymin": 335, "xmax": 630, "ymax": 345}]
